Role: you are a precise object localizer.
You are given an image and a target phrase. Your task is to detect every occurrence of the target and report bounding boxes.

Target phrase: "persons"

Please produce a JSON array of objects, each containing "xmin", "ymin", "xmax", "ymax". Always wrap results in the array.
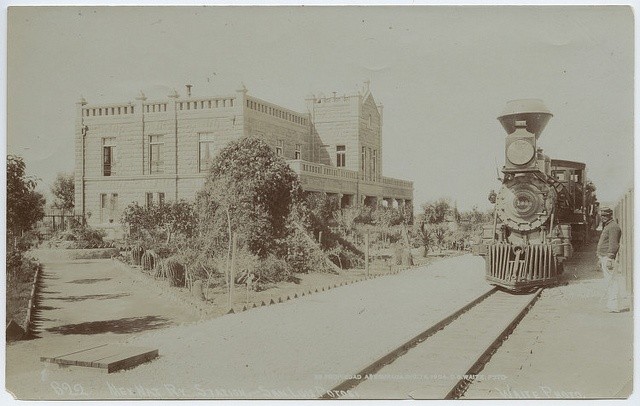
[{"xmin": 595, "ymin": 206, "xmax": 630, "ymax": 313}]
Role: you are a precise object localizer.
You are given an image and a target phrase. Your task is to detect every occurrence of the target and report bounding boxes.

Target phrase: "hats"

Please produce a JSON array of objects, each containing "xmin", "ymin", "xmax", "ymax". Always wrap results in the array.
[{"xmin": 601, "ymin": 207, "xmax": 613, "ymax": 215}]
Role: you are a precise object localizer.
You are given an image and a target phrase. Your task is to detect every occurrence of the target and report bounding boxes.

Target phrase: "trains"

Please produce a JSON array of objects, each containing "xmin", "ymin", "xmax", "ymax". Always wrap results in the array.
[{"xmin": 483, "ymin": 96, "xmax": 600, "ymax": 295}]
